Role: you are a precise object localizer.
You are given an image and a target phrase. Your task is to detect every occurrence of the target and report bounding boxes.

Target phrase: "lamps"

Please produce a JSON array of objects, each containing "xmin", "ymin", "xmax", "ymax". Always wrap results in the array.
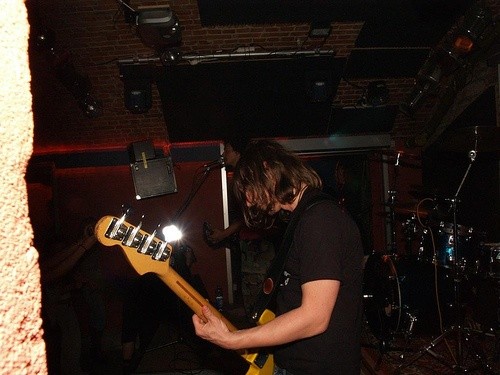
[
  {"xmin": 134, "ymin": 3, "xmax": 184, "ymax": 62},
  {"xmin": 364, "ymin": 79, "xmax": 391, "ymax": 106},
  {"xmin": 398, "ymin": 3, "xmax": 494, "ymax": 120},
  {"xmin": 120, "ymin": 66, "xmax": 154, "ymax": 113}
]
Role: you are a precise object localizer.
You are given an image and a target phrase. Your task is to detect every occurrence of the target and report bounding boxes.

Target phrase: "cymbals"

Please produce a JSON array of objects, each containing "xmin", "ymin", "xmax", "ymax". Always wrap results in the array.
[
  {"xmin": 411, "ymin": 183, "xmax": 454, "ymax": 198},
  {"xmin": 381, "ymin": 203, "xmax": 423, "ymax": 211},
  {"xmin": 378, "ymin": 150, "xmax": 418, "ymax": 158}
]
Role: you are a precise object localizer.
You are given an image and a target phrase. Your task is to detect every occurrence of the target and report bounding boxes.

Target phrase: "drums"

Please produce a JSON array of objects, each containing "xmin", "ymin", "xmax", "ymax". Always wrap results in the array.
[
  {"xmin": 419, "ymin": 220, "xmax": 485, "ymax": 273},
  {"xmin": 366, "ymin": 252, "xmax": 463, "ymax": 335},
  {"xmin": 479, "ymin": 242, "xmax": 500, "ymax": 282}
]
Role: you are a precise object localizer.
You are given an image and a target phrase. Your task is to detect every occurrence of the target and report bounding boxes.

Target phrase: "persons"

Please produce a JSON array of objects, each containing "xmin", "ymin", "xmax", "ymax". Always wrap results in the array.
[
  {"xmin": 205, "ymin": 131, "xmax": 285, "ymax": 320},
  {"xmin": 192, "ymin": 140, "xmax": 362, "ymax": 375},
  {"xmin": 36, "ymin": 221, "xmax": 192, "ymax": 375}
]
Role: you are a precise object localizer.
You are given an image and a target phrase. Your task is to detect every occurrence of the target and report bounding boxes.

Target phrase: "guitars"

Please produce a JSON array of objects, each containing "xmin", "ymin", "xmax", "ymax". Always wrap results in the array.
[
  {"xmin": 95, "ymin": 204, "xmax": 276, "ymax": 375},
  {"xmin": 203, "ymin": 222, "xmax": 239, "ymax": 246}
]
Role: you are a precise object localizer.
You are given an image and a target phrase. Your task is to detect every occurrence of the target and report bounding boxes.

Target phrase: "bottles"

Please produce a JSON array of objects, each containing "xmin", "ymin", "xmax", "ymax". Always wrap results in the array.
[{"xmin": 215, "ymin": 287, "xmax": 225, "ymax": 312}]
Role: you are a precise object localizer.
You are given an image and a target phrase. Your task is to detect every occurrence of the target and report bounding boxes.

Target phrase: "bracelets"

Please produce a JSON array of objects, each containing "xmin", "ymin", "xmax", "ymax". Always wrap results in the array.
[{"xmin": 80, "ymin": 244, "xmax": 88, "ymax": 250}]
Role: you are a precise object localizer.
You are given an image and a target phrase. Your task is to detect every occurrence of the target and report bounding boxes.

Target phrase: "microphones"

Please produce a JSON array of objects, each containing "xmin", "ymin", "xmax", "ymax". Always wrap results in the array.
[{"xmin": 204, "ymin": 156, "xmax": 225, "ymax": 170}]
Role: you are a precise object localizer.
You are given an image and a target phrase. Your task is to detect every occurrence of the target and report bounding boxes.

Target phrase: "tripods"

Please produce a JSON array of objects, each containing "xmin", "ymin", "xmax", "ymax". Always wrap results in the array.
[
  {"xmin": 142, "ymin": 169, "xmax": 212, "ymax": 364},
  {"xmin": 359, "ymin": 146, "xmax": 500, "ymax": 375}
]
